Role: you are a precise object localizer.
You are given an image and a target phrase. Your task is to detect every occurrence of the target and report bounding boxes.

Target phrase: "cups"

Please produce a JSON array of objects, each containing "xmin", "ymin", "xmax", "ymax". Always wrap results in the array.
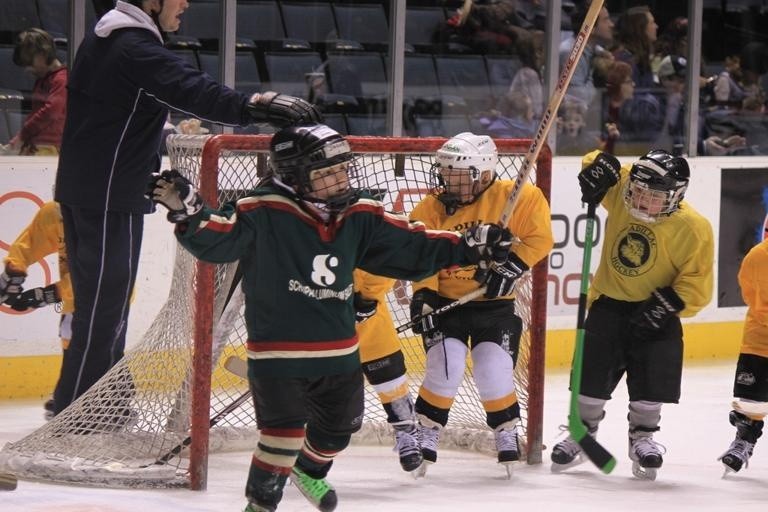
[{"xmin": 306, "ymin": 73, "xmax": 328, "ymax": 102}]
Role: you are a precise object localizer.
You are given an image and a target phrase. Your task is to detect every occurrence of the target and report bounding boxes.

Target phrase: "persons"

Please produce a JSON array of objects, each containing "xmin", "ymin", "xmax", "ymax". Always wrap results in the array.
[
  {"xmin": 550, "ymin": 147, "xmax": 714, "ymax": 469},
  {"xmin": 723, "ymin": 239, "xmax": 767, "ymax": 471},
  {"xmin": 53, "ymin": 0, "xmax": 325, "ymax": 437},
  {"xmin": 351, "ymin": 265, "xmax": 426, "ymax": 472},
  {"xmin": 146, "ymin": 123, "xmax": 510, "ymax": 512},
  {"xmin": 0, "ymin": 174, "xmax": 138, "ymax": 415},
  {"xmin": 408, "ymin": 130, "xmax": 555, "ymax": 461},
  {"xmin": 8, "ymin": 26, "xmax": 72, "ymax": 155},
  {"xmin": 446, "ymin": 0, "xmax": 768, "ymax": 154}
]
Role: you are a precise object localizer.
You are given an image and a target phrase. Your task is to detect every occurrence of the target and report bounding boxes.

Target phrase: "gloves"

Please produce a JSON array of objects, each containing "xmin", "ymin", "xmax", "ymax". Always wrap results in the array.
[
  {"xmin": 459, "ymin": 220, "xmax": 514, "ymax": 269},
  {"xmin": 471, "ymin": 251, "xmax": 531, "ymax": 299},
  {"xmin": 626, "ymin": 283, "xmax": 682, "ymax": 343},
  {"xmin": 244, "ymin": 91, "xmax": 325, "ymax": 130},
  {"xmin": 576, "ymin": 151, "xmax": 621, "ymax": 211},
  {"xmin": 350, "ymin": 288, "xmax": 378, "ymax": 326},
  {"xmin": 142, "ymin": 164, "xmax": 204, "ymax": 223},
  {"xmin": 6, "ymin": 284, "xmax": 62, "ymax": 311},
  {"xmin": 408, "ymin": 286, "xmax": 443, "ymax": 336},
  {"xmin": 0, "ymin": 268, "xmax": 29, "ymax": 304}
]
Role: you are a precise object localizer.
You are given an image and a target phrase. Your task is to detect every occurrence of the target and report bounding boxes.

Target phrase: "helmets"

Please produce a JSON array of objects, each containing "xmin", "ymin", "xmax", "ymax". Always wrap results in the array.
[
  {"xmin": 433, "ymin": 129, "xmax": 501, "ymax": 194},
  {"xmin": 269, "ymin": 122, "xmax": 364, "ymax": 209},
  {"xmin": 625, "ymin": 147, "xmax": 692, "ymax": 216}
]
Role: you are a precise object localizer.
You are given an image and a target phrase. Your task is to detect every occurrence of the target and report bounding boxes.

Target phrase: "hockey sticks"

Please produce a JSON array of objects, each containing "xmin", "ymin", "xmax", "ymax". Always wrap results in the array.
[
  {"xmin": 224, "ymin": 286, "xmax": 489, "ymax": 381},
  {"xmin": 568, "ymin": 205, "xmax": 616, "ymax": 475},
  {"xmin": 109, "ymin": 389, "xmax": 251, "ymax": 468},
  {"xmin": 438, "ymin": 1, "xmax": 605, "ymax": 292}
]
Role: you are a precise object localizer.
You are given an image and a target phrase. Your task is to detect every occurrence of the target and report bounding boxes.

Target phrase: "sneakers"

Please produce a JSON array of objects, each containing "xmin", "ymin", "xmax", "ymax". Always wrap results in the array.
[
  {"xmin": 627, "ymin": 408, "xmax": 666, "ymax": 482},
  {"xmin": 40, "ymin": 393, "xmax": 142, "ymax": 433}
]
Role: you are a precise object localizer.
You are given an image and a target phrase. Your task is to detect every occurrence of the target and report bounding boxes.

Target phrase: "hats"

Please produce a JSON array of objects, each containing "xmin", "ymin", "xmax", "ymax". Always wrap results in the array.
[{"xmin": 654, "ymin": 54, "xmax": 691, "ymax": 79}]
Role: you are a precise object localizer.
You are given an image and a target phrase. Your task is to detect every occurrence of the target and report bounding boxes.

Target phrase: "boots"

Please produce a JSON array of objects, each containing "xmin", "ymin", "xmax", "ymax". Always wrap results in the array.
[
  {"xmin": 387, "ymin": 415, "xmax": 439, "ymax": 480},
  {"xmin": 549, "ymin": 410, "xmax": 606, "ymax": 475},
  {"xmin": 285, "ymin": 460, "xmax": 337, "ymax": 512},
  {"xmin": 493, "ymin": 422, "xmax": 524, "ymax": 481},
  {"xmin": 717, "ymin": 410, "xmax": 764, "ymax": 479}
]
